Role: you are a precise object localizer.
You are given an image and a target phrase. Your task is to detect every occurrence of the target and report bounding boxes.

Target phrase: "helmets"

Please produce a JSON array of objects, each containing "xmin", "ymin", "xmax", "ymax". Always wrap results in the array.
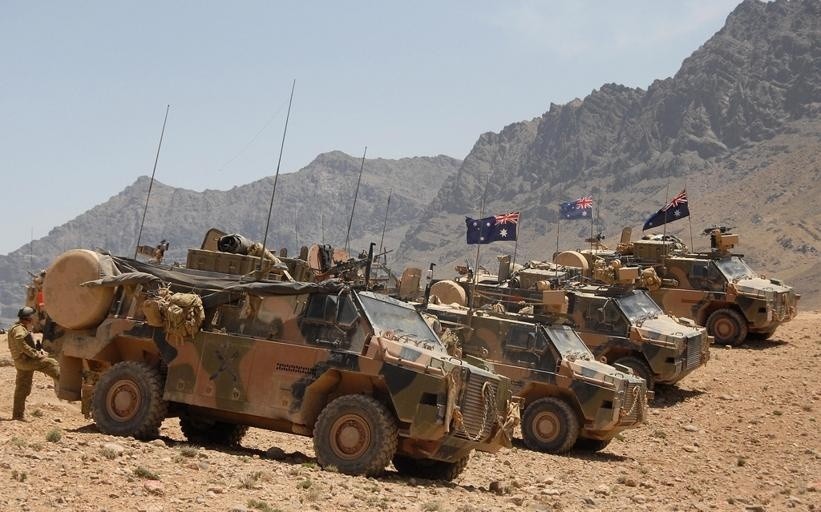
[{"xmin": 18, "ymin": 306, "xmax": 36, "ymax": 317}]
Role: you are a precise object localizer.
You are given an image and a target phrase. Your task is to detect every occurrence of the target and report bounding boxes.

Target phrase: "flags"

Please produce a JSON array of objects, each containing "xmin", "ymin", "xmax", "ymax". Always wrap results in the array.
[
  {"xmin": 465, "ymin": 211, "xmax": 520, "ymax": 243},
  {"xmin": 559, "ymin": 192, "xmax": 594, "ymax": 221},
  {"xmin": 642, "ymin": 187, "xmax": 690, "ymax": 232}
]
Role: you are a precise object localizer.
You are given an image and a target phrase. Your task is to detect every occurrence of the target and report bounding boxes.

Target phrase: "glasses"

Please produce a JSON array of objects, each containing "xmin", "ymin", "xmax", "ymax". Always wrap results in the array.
[{"xmin": 23, "ymin": 307, "xmax": 36, "ymax": 316}]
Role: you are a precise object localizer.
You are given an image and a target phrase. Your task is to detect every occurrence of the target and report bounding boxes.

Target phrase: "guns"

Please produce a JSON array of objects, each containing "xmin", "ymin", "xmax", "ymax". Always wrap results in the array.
[
  {"xmin": 592, "ymin": 232, "xmax": 604, "ymax": 241},
  {"xmin": 35, "ymin": 340, "xmax": 47, "ymax": 378},
  {"xmin": 314, "ymin": 247, "xmax": 393, "ymax": 282},
  {"xmin": 704, "ymin": 226, "xmax": 736, "ymax": 237}
]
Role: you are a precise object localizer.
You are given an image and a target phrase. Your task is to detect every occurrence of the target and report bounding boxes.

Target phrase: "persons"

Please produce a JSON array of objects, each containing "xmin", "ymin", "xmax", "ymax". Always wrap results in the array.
[{"xmin": 6, "ymin": 303, "xmax": 60, "ymax": 425}]
